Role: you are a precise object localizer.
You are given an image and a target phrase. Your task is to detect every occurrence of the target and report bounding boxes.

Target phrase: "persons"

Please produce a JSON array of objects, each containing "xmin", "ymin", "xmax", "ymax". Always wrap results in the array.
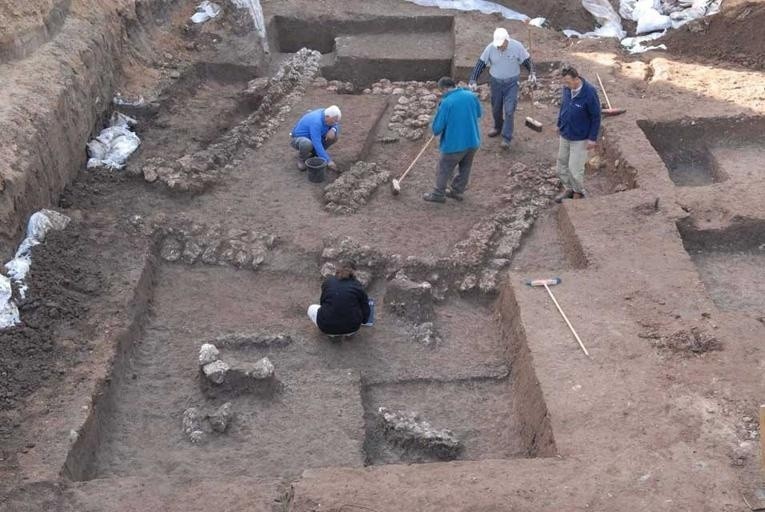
[
  {"xmin": 469, "ymin": 27, "xmax": 536, "ymax": 149},
  {"xmin": 423, "ymin": 77, "xmax": 482, "ymax": 203},
  {"xmin": 554, "ymin": 66, "xmax": 601, "ymax": 202},
  {"xmin": 307, "ymin": 258, "xmax": 371, "ymax": 343},
  {"xmin": 289, "ymin": 104, "xmax": 342, "ymax": 173}
]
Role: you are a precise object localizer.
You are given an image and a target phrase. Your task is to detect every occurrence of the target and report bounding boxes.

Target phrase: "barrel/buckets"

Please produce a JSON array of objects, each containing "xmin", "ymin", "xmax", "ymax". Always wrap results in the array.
[
  {"xmin": 305, "ymin": 156, "xmax": 327, "ymax": 182},
  {"xmin": 361, "ymin": 299, "xmax": 375, "ymax": 327}
]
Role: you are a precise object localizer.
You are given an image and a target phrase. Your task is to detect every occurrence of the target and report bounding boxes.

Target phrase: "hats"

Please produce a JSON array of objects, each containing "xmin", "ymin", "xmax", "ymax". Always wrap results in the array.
[{"xmin": 493, "ymin": 28, "xmax": 509, "ymax": 48}]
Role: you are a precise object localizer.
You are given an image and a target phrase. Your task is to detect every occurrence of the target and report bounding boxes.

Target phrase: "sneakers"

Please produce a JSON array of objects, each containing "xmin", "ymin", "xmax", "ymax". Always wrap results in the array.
[
  {"xmin": 501, "ymin": 138, "xmax": 510, "ymax": 149},
  {"xmin": 446, "ymin": 187, "xmax": 463, "ymax": 200},
  {"xmin": 488, "ymin": 126, "xmax": 502, "ymax": 136},
  {"xmin": 422, "ymin": 190, "xmax": 445, "ymax": 202},
  {"xmin": 297, "ymin": 159, "xmax": 305, "ymax": 170},
  {"xmin": 555, "ymin": 189, "xmax": 582, "ymax": 202}
]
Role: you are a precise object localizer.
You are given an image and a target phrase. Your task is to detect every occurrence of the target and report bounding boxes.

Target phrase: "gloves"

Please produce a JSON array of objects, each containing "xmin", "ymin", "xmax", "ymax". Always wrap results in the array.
[
  {"xmin": 468, "ymin": 79, "xmax": 478, "ymax": 92},
  {"xmin": 528, "ymin": 71, "xmax": 536, "ymax": 88}
]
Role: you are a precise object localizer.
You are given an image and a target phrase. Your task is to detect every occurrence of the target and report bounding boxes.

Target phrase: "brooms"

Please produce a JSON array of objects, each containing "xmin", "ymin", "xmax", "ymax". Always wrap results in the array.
[
  {"xmin": 526, "ymin": 277, "xmax": 589, "ymax": 356},
  {"xmin": 391, "ymin": 134, "xmax": 436, "ymax": 195},
  {"xmin": 525, "ymin": 28, "xmax": 543, "ymax": 132},
  {"xmin": 596, "ymin": 73, "xmax": 627, "ymax": 114}
]
